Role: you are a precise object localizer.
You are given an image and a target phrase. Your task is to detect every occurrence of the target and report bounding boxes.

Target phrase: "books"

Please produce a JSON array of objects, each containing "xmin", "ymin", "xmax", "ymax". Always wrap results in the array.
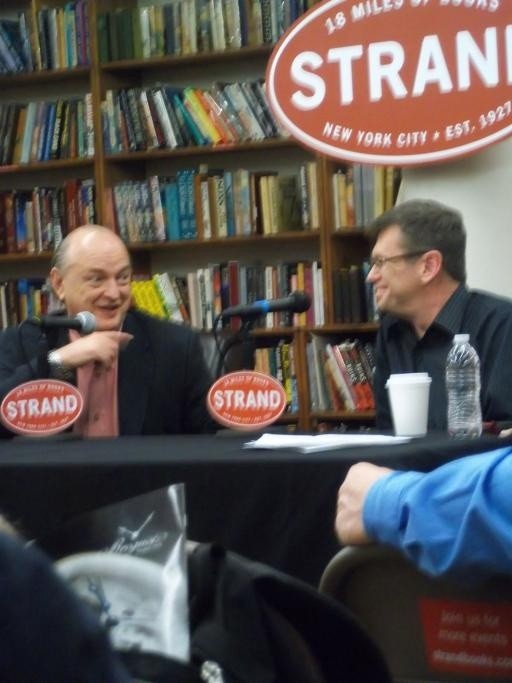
[
  {"xmin": 2, "ymin": 2, "xmax": 89, "ymax": 75},
  {"xmin": 333, "ymin": 162, "xmax": 394, "ymax": 229},
  {"xmin": 306, "ymin": 337, "xmax": 376, "ymax": 410},
  {"xmin": 103, "ymin": 160, "xmax": 318, "ymax": 243},
  {"xmin": 255, "ymin": 341, "xmax": 299, "ymax": 412},
  {"xmin": 1, "ymin": 278, "xmax": 67, "ymax": 331},
  {"xmin": 132, "ymin": 258, "xmax": 324, "ymax": 331},
  {"xmin": 98, "ymin": 0, "xmax": 324, "ymax": 61},
  {"xmin": 0, "ymin": 93, "xmax": 95, "ymax": 165},
  {"xmin": 100, "ymin": 79, "xmax": 293, "ymax": 153},
  {"xmin": 1, "ymin": 179, "xmax": 96, "ymax": 254},
  {"xmin": 331, "ymin": 261, "xmax": 380, "ymax": 322}
]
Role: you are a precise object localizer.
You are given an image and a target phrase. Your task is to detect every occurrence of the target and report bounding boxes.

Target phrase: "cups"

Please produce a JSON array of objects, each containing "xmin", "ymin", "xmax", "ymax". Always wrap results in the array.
[{"xmin": 385, "ymin": 371, "xmax": 433, "ymax": 437}]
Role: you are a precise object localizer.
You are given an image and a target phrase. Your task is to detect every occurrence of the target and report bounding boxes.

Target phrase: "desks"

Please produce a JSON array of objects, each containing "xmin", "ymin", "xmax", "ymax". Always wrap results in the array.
[{"xmin": 0, "ymin": 432, "xmax": 511, "ymax": 573}]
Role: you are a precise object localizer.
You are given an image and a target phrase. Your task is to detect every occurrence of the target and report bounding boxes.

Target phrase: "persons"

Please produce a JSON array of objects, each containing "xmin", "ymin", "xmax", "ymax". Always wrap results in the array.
[
  {"xmin": 333, "ymin": 444, "xmax": 512, "ymax": 585},
  {"xmin": 0, "ymin": 224, "xmax": 217, "ymax": 435},
  {"xmin": 0, "ymin": 516, "xmax": 129, "ymax": 683},
  {"xmin": 359, "ymin": 199, "xmax": 512, "ymax": 430}
]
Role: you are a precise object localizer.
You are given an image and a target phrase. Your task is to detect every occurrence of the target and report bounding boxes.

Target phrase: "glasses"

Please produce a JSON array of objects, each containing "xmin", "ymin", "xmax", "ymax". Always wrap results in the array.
[{"xmin": 371, "ymin": 250, "xmax": 425, "ymax": 267}]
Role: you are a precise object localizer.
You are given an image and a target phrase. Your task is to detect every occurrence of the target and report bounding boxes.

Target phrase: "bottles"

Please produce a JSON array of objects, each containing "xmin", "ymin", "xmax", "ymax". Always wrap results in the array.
[{"xmin": 446, "ymin": 334, "xmax": 484, "ymax": 435}]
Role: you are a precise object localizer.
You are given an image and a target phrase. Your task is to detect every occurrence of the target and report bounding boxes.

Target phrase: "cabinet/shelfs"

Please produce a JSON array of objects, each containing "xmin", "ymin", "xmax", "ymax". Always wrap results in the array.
[{"xmin": 0, "ymin": 1, "xmax": 397, "ymax": 434}]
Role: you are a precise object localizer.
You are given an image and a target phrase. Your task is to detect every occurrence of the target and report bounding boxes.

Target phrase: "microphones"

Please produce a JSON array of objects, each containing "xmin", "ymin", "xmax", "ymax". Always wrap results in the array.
[
  {"xmin": 218, "ymin": 291, "xmax": 311, "ymax": 314},
  {"xmin": 36, "ymin": 311, "xmax": 96, "ymax": 334}
]
